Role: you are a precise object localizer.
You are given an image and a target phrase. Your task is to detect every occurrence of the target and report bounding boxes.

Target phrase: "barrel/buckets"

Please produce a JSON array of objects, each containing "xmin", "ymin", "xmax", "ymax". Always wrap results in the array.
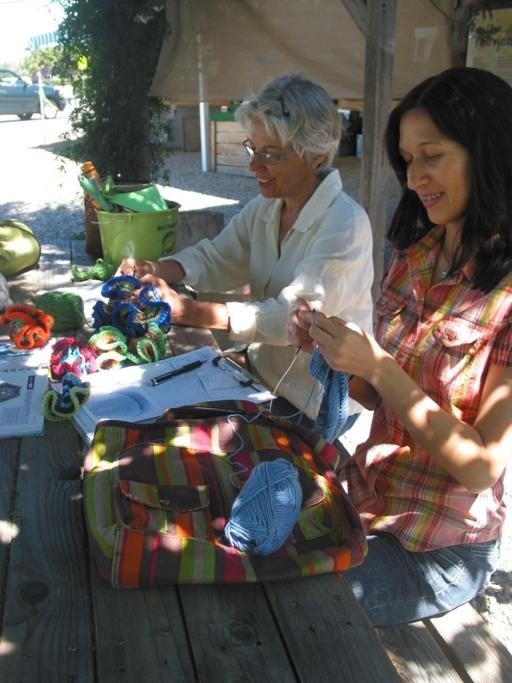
[{"xmin": 94, "ymin": 203, "xmax": 181, "ymax": 275}]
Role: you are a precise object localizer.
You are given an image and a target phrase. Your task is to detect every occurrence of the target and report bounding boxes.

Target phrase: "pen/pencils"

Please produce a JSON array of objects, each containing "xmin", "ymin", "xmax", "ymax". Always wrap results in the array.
[{"xmin": 150, "ymin": 357, "xmax": 211, "ymax": 385}]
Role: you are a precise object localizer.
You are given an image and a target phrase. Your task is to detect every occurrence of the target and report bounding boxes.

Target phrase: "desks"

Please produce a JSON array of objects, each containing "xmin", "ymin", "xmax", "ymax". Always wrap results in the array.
[{"xmin": 0, "ymin": 240, "xmax": 402, "ymax": 683}]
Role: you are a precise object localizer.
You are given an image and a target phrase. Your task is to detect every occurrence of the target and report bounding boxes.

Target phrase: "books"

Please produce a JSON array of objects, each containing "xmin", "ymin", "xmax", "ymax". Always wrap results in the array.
[{"xmin": 0, "ymin": 332, "xmax": 59, "ymax": 440}]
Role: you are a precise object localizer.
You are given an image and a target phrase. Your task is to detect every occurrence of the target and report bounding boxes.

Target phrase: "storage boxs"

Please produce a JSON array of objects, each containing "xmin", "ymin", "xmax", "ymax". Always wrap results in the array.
[{"xmin": 211, "ymin": 120, "xmax": 258, "ymax": 177}]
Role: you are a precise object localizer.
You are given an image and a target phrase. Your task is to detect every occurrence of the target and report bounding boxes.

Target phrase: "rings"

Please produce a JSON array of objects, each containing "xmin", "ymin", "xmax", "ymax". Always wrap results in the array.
[{"xmin": 314, "ymin": 342, "xmax": 320, "ymax": 353}]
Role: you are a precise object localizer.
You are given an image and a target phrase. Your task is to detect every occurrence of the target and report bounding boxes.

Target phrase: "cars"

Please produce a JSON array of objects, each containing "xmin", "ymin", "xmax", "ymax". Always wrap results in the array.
[{"xmin": 0, "ymin": 68, "xmax": 66, "ymax": 119}]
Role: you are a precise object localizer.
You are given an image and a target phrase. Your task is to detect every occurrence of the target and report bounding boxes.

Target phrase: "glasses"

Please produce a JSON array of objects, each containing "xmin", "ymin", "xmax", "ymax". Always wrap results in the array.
[{"xmin": 241, "ymin": 138, "xmax": 295, "ymax": 166}]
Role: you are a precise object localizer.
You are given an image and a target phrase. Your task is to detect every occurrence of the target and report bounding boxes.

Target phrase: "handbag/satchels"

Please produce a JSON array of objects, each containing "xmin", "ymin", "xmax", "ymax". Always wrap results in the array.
[
  {"xmin": 0, "ymin": 218, "xmax": 41, "ymax": 277},
  {"xmin": 82, "ymin": 398, "xmax": 369, "ymax": 592}
]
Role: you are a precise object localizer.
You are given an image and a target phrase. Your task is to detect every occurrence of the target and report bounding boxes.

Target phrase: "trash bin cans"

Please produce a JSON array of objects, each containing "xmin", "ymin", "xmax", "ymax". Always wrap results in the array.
[{"xmin": 84, "ymin": 183, "xmax": 181, "ymax": 263}]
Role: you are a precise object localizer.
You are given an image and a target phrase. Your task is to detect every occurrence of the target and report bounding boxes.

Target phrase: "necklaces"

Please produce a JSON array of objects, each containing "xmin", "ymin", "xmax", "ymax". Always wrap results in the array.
[{"xmin": 437, "ymin": 250, "xmax": 451, "ymax": 278}]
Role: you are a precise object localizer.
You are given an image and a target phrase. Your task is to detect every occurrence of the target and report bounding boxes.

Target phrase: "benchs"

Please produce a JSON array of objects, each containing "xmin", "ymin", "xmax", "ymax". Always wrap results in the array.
[{"xmin": 371, "ymin": 601, "xmax": 511, "ymax": 683}]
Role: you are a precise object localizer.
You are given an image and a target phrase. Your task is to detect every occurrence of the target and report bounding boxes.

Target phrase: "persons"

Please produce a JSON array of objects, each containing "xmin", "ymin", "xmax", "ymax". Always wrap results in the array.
[
  {"xmin": 117, "ymin": 73, "xmax": 376, "ymax": 429},
  {"xmin": 283, "ymin": 66, "xmax": 512, "ymax": 630}
]
